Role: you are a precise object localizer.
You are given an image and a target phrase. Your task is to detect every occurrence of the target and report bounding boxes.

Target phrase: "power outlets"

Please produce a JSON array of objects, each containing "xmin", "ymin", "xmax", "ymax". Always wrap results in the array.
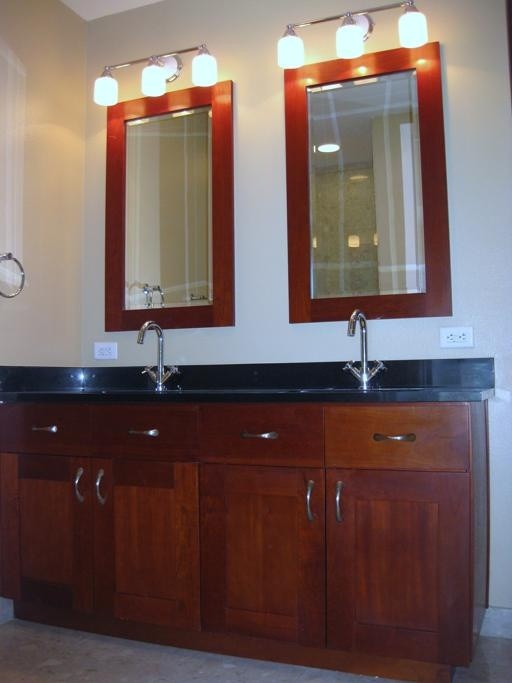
[{"xmin": 439, "ymin": 325, "xmax": 474, "ymax": 349}]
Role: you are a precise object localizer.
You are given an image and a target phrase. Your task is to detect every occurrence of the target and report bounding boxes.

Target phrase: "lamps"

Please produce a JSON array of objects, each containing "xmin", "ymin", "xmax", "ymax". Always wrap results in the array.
[
  {"xmin": 92, "ymin": 40, "xmax": 218, "ymax": 108},
  {"xmin": 276, "ymin": 0, "xmax": 430, "ymax": 69}
]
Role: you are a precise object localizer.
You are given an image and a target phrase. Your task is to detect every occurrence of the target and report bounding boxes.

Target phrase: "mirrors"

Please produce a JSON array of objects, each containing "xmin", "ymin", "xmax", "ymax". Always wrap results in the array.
[
  {"xmin": 103, "ymin": 78, "xmax": 237, "ymax": 330},
  {"xmin": 285, "ymin": 41, "xmax": 454, "ymax": 325}
]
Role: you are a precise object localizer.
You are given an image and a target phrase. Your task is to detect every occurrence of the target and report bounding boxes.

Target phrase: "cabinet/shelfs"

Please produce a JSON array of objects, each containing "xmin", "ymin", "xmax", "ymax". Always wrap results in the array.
[
  {"xmin": 201, "ymin": 403, "xmax": 492, "ymax": 681},
  {"xmin": 0, "ymin": 402, "xmax": 201, "ymax": 651}
]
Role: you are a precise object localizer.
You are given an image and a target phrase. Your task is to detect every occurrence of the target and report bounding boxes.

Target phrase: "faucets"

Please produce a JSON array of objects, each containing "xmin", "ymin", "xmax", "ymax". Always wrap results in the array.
[
  {"xmin": 346, "ymin": 309, "xmax": 369, "ymax": 386},
  {"xmin": 137, "ymin": 319, "xmax": 165, "ymax": 388}
]
